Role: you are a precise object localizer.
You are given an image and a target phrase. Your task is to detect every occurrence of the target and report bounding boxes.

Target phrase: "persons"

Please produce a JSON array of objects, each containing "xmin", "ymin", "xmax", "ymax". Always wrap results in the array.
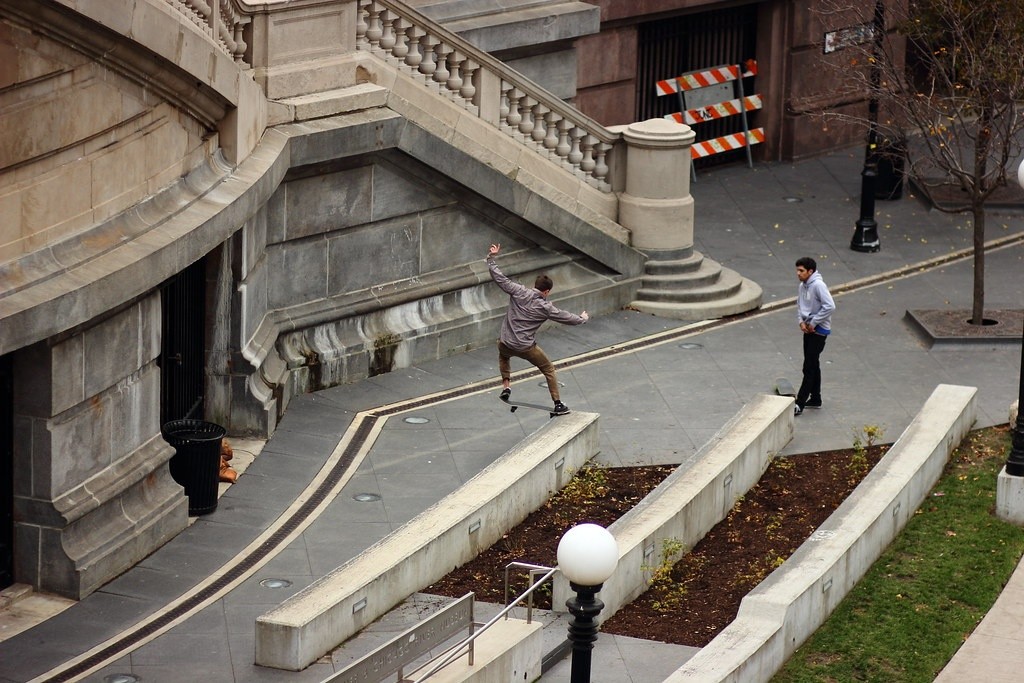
[
  {"xmin": 487, "ymin": 244, "xmax": 588, "ymax": 414},
  {"xmin": 794, "ymin": 256, "xmax": 836, "ymax": 416}
]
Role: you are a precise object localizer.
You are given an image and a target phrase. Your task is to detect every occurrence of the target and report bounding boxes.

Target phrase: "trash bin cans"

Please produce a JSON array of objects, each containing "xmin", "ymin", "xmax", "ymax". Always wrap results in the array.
[
  {"xmin": 161, "ymin": 419, "xmax": 227, "ymax": 517},
  {"xmin": 865, "ymin": 135, "xmax": 907, "ymax": 199}
]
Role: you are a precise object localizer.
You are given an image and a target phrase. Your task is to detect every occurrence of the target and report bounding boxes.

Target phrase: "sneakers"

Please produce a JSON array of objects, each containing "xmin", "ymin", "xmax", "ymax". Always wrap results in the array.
[
  {"xmin": 554, "ymin": 403, "xmax": 569, "ymax": 414},
  {"xmin": 499, "ymin": 387, "xmax": 511, "ymax": 401},
  {"xmin": 794, "ymin": 399, "xmax": 804, "ymax": 415},
  {"xmin": 804, "ymin": 396, "xmax": 822, "ymax": 408}
]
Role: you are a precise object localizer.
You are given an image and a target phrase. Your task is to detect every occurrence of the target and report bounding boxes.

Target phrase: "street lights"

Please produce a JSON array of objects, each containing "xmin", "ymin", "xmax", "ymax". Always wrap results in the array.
[
  {"xmin": 553, "ymin": 520, "xmax": 617, "ymax": 683},
  {"xmin": 996, "ymin": 160, "xmax": 1024, "ymax": 527}
]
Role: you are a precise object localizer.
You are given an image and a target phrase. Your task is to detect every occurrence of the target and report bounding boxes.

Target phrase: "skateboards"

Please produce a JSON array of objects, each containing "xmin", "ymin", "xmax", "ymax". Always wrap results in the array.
[
  {"xmin": 775, "ymin": 377, "xmax": 797, "ymax": 396},
  {"xmin": 497, "ymin": 396, "xmax": 572, "ymax": 419}
]
